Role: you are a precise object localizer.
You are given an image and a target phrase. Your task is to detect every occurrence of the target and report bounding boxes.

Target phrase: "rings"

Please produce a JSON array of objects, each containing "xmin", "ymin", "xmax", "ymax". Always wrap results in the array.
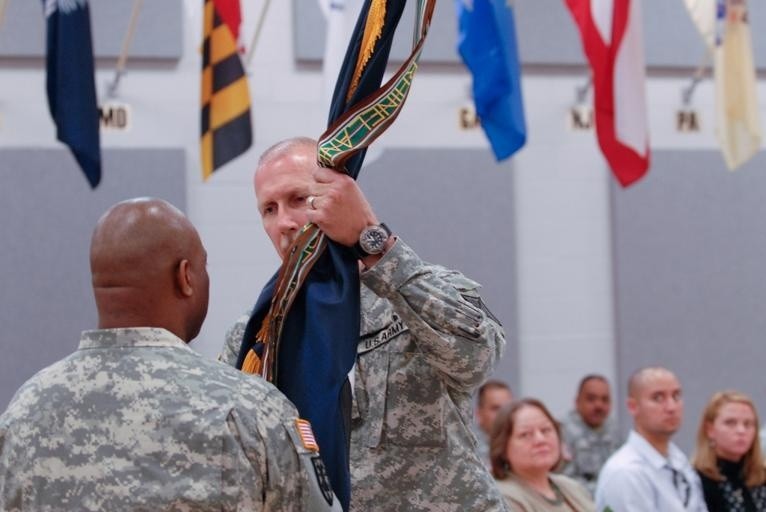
[{"xmin": 307, "ymin": 197, "xmax": 316, "ymax": 210}]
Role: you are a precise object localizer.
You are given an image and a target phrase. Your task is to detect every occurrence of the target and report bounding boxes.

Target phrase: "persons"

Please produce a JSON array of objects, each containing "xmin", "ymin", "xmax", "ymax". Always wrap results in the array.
[
  {"xmin": 217, "ymin": 136, "xmax": 512, "ymax": 512},
  {"xmin": 593, "ymin": 364, "xmax": 711, "ymax": 511},
  {"xmin": 471, "ymin": 378, "xmax": 511, "ymax": 470},
  {"xmin": 493, "ymin": 397, "xmax": 600, "ymax": 511},
  {"xmin": 0, "ymin": 194, "xmax": 348, "ymax": 512},
  {"xmin": 557, "ymin": 373, "xmax": 620, "ymax": 487},
  {"xmin": 695, "ymin": 390, "xmax": 766, "ymax": 512}
]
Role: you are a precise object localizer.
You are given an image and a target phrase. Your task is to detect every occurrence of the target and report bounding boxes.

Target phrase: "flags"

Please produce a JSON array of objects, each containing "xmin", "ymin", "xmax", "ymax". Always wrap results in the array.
[
  {"xmin": 42, "ymin": 0, "xmax": 99, "ymax": 191},
  {"xmin": 200, "ymin": 0, "xmax": 253, "ymax": 180},
  {"xmin": 234, "ymin": 0, "xmax": 413, "ymax": 512},
  {"xmin": 684, "ymin": 2, "xmax": 765, "ymax": 172},
  {"xmin": 450, "ymin": 2, "xmax": 532, "ymax": 161},
  {"xmin": 567, "ymin": 2, "xmax": 652, "ymax": 188}
]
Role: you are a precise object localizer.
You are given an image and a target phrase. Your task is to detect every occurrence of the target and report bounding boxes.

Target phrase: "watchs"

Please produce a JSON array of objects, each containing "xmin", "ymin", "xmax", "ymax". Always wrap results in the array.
[{"xmin": 354, "ymin": 222, "xmax": 392, "ymax": 256}]
